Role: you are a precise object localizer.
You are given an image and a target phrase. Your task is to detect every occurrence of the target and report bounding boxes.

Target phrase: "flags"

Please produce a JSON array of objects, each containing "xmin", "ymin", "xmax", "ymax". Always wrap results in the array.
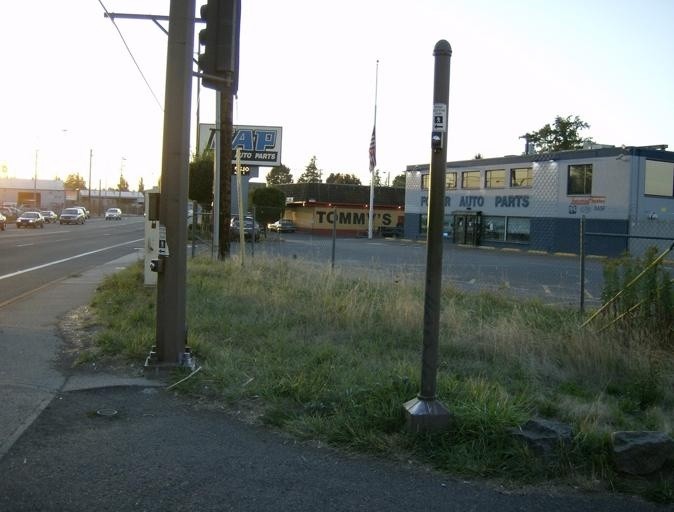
[{"xmin": 369, "ymin": 126, "xmax": 376, "ymax": 172}]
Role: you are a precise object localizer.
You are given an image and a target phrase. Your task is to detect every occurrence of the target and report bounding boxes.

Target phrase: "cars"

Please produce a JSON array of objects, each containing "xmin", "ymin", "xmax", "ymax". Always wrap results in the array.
[
  {"xmin": 104, "ymin": 208, "xmax": 122, "ymax": 221},
  {"xmin": 230, "ymin": 216, "xmax": 296, "ymax": 241},
  {"xmin": 0, "ymin": 204, "xmax": 89, "ymax": 230}
]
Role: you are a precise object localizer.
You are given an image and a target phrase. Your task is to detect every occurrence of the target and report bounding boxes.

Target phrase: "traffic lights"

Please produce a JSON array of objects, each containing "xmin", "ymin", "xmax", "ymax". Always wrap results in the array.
[{"xmin": 199, "ymin": 0, "xmax": 241, "ymax": 94}]
